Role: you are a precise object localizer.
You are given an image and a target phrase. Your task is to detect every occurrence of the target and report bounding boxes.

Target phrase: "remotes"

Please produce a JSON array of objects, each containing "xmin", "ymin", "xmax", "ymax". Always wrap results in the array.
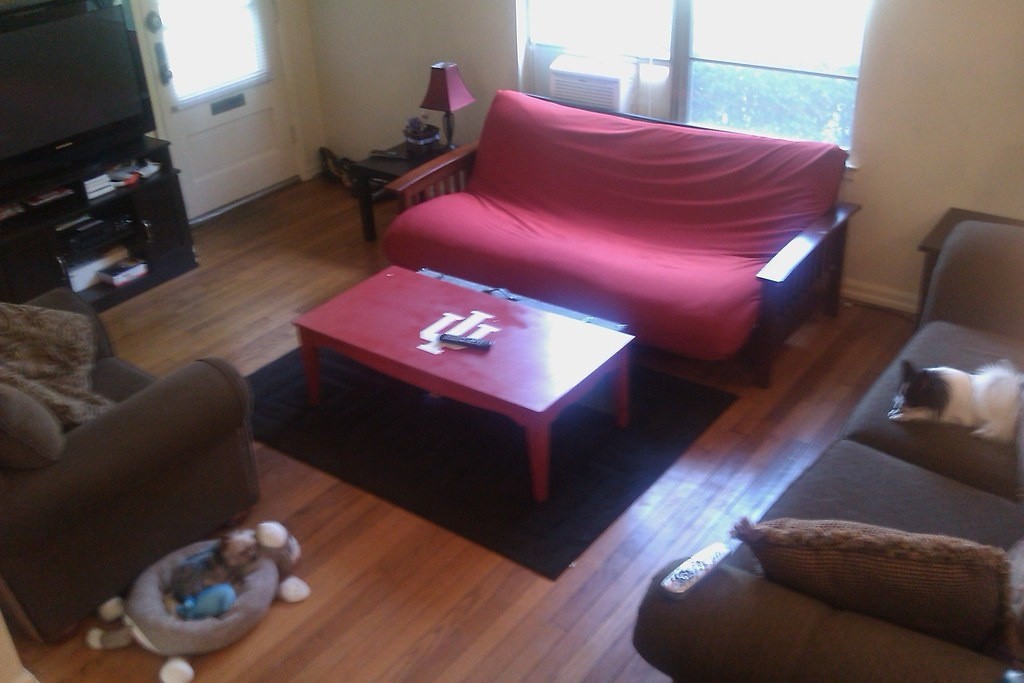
[
  {"xmin": 367, "ymin": 150, "xmax": 409, "ymax": 160},
  {"xmin": 438, "ymin": 329, "xmax": 495, "ymax": 351}
]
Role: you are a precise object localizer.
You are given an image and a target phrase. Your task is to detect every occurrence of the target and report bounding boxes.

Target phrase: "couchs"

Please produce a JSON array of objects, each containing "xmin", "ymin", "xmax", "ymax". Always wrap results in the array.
[
  {"xmin": 0, "ymin": 286, "xmax": 262, "ymax": 643},
  {"xmin": 378, "ymin": 89, "xmax": 863, "ymax": 388},
  {"xmin": 631, "ymin": 220, "xmax": 1024, "ymax": 683}
]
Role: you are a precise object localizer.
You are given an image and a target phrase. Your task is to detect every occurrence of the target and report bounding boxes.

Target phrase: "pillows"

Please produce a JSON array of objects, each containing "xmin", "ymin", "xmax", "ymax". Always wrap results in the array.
[{"xmin": 729, "ymin": 514, "xmax": 1022, "ymax": 673}]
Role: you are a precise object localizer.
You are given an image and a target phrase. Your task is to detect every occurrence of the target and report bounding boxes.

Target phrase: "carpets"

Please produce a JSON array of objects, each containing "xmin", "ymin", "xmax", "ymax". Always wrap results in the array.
[{"xmin": 240, "ymin": 345, "xmax": 741, "ymax": 583}]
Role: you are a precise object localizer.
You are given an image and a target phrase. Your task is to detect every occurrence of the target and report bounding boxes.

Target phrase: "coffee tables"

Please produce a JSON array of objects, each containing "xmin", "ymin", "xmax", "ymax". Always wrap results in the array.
[{"xmin": 291, "ymin": 264, "xmax": 636, "ymax": 504}]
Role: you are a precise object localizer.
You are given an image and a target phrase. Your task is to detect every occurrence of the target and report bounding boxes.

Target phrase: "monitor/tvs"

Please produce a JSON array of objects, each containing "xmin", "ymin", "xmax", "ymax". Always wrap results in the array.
[{"xmin": 0, "ymin": 0, "xmax": 156, "ymax": 187}]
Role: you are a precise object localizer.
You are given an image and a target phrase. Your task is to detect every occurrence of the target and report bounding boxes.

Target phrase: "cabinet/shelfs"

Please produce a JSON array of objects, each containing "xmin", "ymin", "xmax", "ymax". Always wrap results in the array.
[{"xmin": 1, "ymin": 135, "xmax": 200, "ymax": 313}]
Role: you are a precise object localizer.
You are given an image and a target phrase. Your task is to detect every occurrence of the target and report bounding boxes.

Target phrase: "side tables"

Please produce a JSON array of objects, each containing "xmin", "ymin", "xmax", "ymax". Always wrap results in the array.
[
  {"xmin": 358, "ymin": 139, "xmax": 464, "ymax": 243},
  {"xmin": 915, "ymin": 208, "xmax": 1024, "ymax": 327}
]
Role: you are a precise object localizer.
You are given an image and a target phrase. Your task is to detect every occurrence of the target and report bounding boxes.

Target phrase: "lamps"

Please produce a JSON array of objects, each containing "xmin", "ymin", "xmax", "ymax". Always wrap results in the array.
[{"xmin": 418, "ymin": 61, "xmax": 479, "ymax": 151}]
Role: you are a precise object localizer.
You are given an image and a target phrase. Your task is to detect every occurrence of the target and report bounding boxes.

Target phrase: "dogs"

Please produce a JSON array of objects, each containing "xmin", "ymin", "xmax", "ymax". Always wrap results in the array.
[{"xmin": 887, "ymin": 358, "xmax": 1024, "ymax": 443}]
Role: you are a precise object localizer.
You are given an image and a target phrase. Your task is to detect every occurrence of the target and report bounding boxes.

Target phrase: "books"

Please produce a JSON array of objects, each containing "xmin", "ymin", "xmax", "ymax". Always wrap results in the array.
[{"xmin": 0, "ymin": 159, "xmax": 161, "ymax": 294}]
